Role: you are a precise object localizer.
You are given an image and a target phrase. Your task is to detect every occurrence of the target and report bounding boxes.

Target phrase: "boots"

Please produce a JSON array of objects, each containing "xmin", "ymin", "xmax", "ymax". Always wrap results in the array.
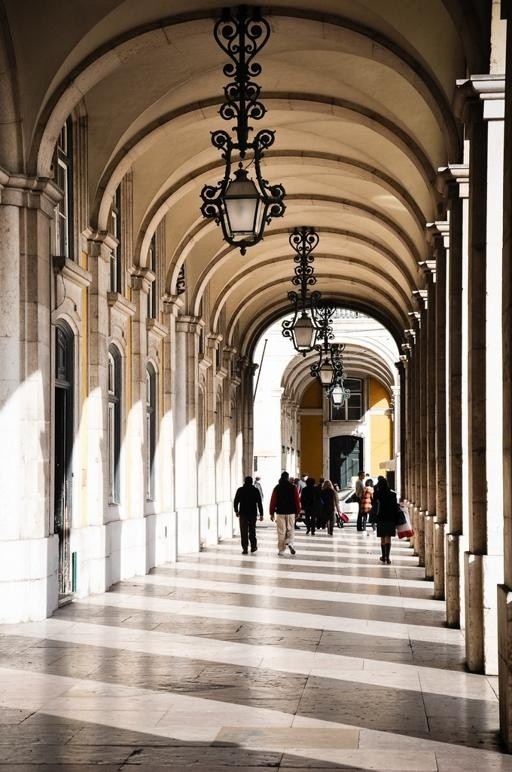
[
  {"xmin": 363, "ymin": 521, "xmax": 366, "ymax": 531},
  {"xmin": 380, "ymin": 544, "xmax": 386, "ymax": 562},
  {"xmin": 372, "ymin": 524, "xmax": 377, "ymax": 531},
  {"xmin": 385, "ymin": 543, "xmax": 391, "ymax": 564}
]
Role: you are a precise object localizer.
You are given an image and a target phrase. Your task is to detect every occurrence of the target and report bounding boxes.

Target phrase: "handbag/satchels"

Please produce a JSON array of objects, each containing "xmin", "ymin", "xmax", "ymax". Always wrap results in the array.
[
  {"xmin": 396, "ymin": 506, "xmax": 406, "ymax": 528},
  {"xmin": 339, "ymin": 512, "xmax": 349, "ymax": 522},
  {"xmin": 398, "ymin": 506, "xmax": 414, "ymax": 539}
]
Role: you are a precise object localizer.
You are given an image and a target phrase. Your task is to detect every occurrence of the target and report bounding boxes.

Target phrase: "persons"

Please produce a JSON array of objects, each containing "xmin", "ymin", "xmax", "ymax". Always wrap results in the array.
[
  {"xmin": 233, "ymin": 477, "xmax": 263, "ymax": 554},
  {"xmin": 370, "ymin": 477, "xmax": 399, "ymax": 563},
  {"xmin": 289, "ymin": 472, "xmax": 342, "ymax": 536},
  {"xmin": 269, "ymin": 471, "xmax": 301, "ymax": 555},
  {"xmin": 254, "ymin": 476, "xmax": 264, "ymax": 517},
  {"xmin": 355, "ymin": 472, "xmax": 366, "ymax": 532},
  {"xmin": 360, "ymin": 479, "xmax": 377, "ymax": 536},
  {"xmin": 371, "ymin": 476, "xmax": 385, "ymax": 534}
]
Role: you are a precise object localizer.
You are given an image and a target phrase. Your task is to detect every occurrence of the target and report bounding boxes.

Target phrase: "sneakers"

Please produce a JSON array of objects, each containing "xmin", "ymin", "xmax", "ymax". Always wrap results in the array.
[{"xmin": 288, "ymin": 545, "xmax": 295, "ymax": 555}]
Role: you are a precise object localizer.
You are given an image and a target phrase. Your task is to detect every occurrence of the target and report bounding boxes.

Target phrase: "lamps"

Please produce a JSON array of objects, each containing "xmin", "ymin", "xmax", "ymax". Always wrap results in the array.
[
  {"xmin": 282, "ymin": 225, "xmax": 351, "ymax": 410},
  {"xmin": 200, "ymin": 6, "xmax": 287, "ymax": 256}
]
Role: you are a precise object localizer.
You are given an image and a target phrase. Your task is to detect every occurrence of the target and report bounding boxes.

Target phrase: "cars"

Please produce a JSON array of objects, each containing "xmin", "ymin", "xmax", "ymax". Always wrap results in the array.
[{"xmin": 299, "ymin": 486, "xmax": 396, "ymax": 524}]
